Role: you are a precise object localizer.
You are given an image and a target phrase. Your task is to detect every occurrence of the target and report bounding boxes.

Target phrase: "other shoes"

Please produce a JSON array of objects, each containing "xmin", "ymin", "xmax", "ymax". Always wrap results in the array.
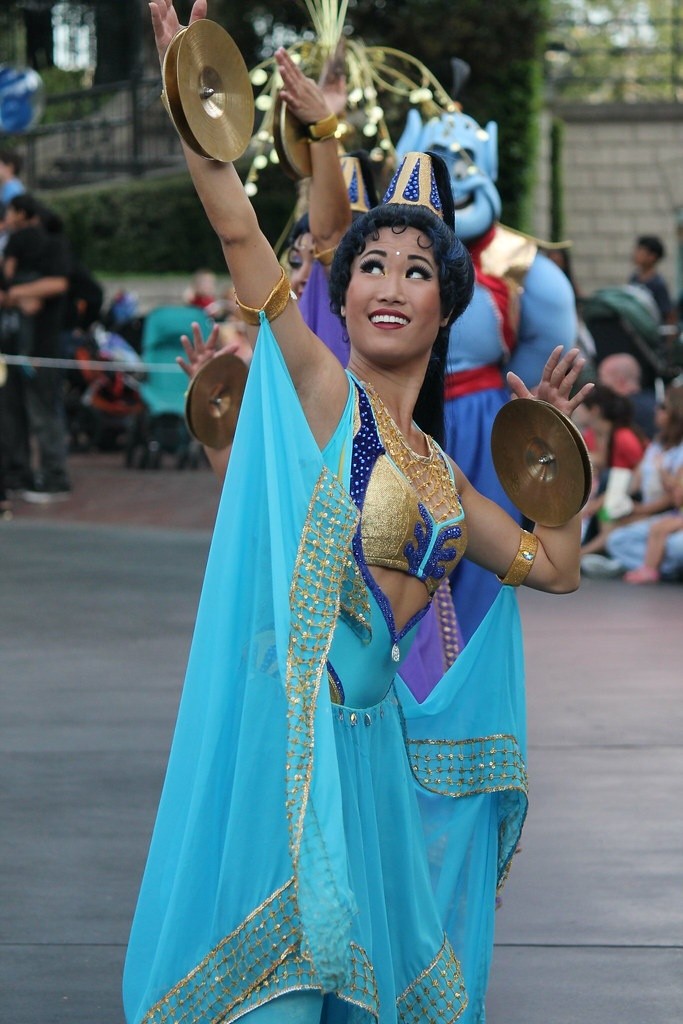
[{"xmin": 23, "ymin": 471, "xmax": 73, "ymax": 504}]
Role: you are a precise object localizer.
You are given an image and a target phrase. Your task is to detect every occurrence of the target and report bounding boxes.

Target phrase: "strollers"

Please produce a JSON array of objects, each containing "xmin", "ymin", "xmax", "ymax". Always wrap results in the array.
[
  {"xmin": 126, "ymin": 305, "xmax": 215, "ymax": 472},
  {"xmin": 64, "ymin": 322, "xmax": 144, "ymax": 452}
]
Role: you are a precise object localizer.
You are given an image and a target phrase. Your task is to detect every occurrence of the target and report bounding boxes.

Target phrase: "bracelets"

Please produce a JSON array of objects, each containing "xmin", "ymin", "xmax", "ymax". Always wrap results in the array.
[{"xmin": 307, "ymin": 113, "xmax": 339, "ymax": 141}]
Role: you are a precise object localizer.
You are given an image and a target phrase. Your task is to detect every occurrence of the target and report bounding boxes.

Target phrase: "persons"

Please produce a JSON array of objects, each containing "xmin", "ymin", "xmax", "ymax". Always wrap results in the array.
[
  {"xmin": 265, "ymin": 50, "xmax": 475, "ymax": 703},
  {"xmin": 581, "ymin": 234, "xmax": 683, "ymax": 585},
  {"xmin": 122, "ymin": 0, "xmax": 595, "ymax": 1024},
  {"xmin": 392, "ymin": 109, "xmax": 577, "ymax": 644},
  {"xmin": 1, "ymin": 140, "xmax": 104, "ymax": 503}
]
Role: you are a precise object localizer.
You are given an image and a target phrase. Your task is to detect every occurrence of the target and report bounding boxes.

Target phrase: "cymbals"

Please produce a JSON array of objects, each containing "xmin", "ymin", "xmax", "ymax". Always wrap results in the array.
[
  {"xmin": 182, "ymin": 351, "xmax": 250, "ymax": 450},
  {"xmin": 160, "ymin": 15, "xmax": 258, "ymax": 164},
  {"xmin": 488, "ymin": 396, "xmax": 594, "ymax": 531},
  {"xmin": 269, "ymin": 90, "xmax": 315, "ymax": 183}
]
